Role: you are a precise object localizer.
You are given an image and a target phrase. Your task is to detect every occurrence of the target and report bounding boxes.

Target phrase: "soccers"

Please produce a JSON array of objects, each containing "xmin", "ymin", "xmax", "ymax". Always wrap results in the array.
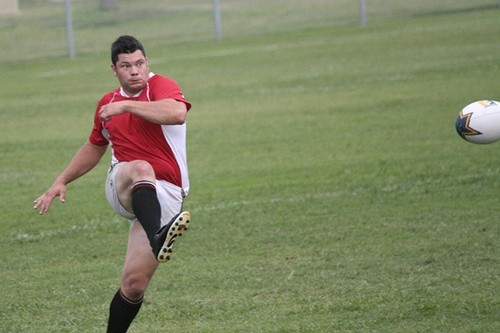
[{"xmin": 455, "ymin": 99, "xmax": 500, "ymax": 143}]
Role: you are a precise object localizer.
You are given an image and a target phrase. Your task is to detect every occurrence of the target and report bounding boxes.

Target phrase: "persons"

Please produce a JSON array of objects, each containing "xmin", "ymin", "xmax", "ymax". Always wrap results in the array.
[{"xmin": 32, "ymin": 35, "xmax": 192, "ymax": 332}]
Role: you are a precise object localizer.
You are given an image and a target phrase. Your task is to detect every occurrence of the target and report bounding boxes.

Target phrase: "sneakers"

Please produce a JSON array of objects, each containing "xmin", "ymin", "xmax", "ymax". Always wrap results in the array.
[{"xmin": 150, "ymin": 208, "xmax": 190, "ymax": 263}]
